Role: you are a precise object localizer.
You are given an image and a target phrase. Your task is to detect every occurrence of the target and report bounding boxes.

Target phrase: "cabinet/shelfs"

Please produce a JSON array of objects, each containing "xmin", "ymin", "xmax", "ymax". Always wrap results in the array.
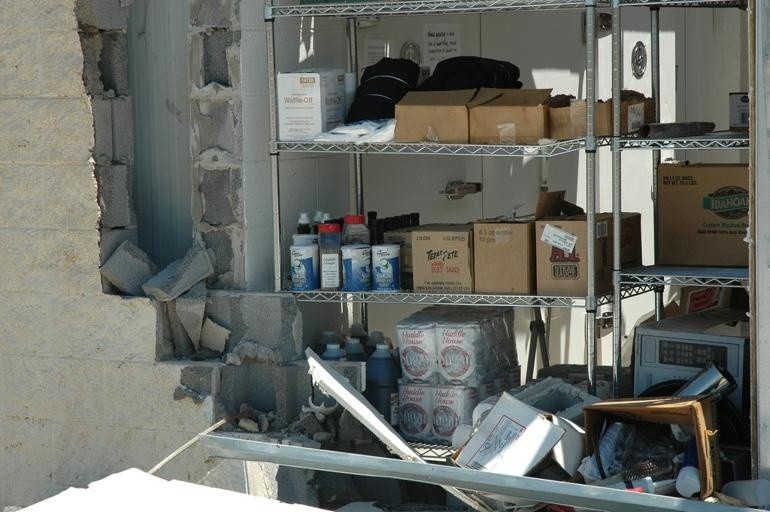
[
  {"xmin": 601, "ymin": 1, "xmax": 762, "ymax": 397},
  {"xmin": 262, "ymin": 2, "xmax": 663, "ymax": 385}
]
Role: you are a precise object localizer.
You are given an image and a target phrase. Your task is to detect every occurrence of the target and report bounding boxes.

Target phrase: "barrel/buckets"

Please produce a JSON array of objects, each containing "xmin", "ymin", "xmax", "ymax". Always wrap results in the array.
[{"xmin": 320, "ymin": 323, "xmax": 401, "ymax": 432}]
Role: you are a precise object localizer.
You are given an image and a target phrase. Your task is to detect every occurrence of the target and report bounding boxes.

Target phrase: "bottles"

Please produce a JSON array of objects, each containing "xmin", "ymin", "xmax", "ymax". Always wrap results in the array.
[
  {"xmin": 317, "ymin": 222, "xmax": 345, "ymax": 291},
  {"xmin": 313, "ymin": 325, "xmax": 399, "ymax": 427},
  {"xmin": 341, "ymin": 214, "xmax": 370, "ymax": 245},
  {"xmin": 297, "ymin": 211, "xmax": 331, "ymax": 235}
]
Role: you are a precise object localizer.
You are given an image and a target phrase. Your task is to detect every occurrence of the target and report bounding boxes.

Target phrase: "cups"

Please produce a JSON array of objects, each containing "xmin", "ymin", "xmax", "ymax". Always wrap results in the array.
[
  {"xmin": 289, "ymin": 242, "xmax": 318, "ymax": 290},
  {"xmin": 372, "ymin": 245, "xmax": 404, "ymax": 296},
  {"xmin": 340, "ymin": 244, "xmax": 370, "ymax": 291}
]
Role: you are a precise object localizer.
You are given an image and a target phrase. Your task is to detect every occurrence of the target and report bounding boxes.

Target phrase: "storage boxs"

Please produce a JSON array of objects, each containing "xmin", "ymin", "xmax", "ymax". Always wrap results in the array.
[
  {"xmin": 534, "ymin": 188, "xmax": 643, "ymax": 300},
  {"xmin": 411, "ymin": 223, "xmax": 472, "ymax": 295},
  {"xmin": 277, "ymin": 65, "xmax": 346, "ymax": 144},
  {"xmin": 447, "ymin": 391, "xmax": 589, "ymax": 510},
  {"xmin": 473, "ymin": 215, "xmax": 534, "ymax": 292},
  {"xmin": 655, "ymin": 161, "xmax": 752, "ymax": 267},
  {"xmin": 585, "ymin": 394, "xmax": 719, "ymax": 500},
  {"xmin": 392, "ymin": 86, "xmax": 655, "ymax": 146}
]
